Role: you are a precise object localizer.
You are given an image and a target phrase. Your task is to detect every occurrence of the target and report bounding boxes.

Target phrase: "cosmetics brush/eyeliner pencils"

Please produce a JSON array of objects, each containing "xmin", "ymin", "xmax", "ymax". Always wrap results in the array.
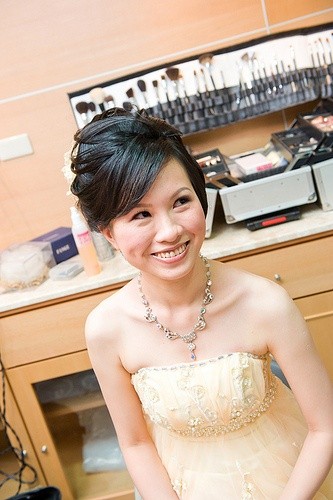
[{"xmin": 76, "ymin": 31, "xmax": 333, "ymax": 124}]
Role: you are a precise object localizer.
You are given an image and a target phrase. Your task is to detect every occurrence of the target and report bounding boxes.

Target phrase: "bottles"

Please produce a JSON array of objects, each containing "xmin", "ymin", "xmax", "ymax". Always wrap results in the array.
[{"xmin": 70, "ymin": 206, "xmax": 115, "ymax": 276}]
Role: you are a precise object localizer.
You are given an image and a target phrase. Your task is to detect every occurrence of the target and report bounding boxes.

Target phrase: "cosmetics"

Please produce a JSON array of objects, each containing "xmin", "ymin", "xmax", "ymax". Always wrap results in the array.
[
  {"xmin": 92, "ymin": 231, "xmax": 116, "ymax": 261},
  {"xmin": 71, "ymin": 207, "xmax": 101, "ymax": 275}
]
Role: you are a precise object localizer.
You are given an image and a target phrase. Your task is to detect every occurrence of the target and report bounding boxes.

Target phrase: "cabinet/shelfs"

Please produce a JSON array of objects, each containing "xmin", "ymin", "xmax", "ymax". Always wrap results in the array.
[{"xmin": 0, "ymin": 231, "xmax": 333, "ymax": 500}]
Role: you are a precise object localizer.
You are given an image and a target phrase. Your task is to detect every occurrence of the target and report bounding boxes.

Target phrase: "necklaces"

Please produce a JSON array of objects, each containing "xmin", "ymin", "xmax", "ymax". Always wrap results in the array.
[{"xmin": 137, "ymin": 252, "xmax": 214, "ymax": 360}]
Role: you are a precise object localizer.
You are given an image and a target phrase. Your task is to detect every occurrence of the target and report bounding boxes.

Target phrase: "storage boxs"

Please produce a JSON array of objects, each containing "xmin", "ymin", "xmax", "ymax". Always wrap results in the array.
[
  {"xmin": 189, "ymin": 98, "xmax": 333, "ymax": 238},
  {"xmin": 31, "ymin": 227, "xmax": 79, "ymax": 264}
]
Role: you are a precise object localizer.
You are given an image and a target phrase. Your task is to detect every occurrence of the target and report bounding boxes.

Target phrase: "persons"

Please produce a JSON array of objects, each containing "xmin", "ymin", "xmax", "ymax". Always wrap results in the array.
[{"xmin": 70, "ymin": 105, "xmax": 333, "ymax": 500}]
